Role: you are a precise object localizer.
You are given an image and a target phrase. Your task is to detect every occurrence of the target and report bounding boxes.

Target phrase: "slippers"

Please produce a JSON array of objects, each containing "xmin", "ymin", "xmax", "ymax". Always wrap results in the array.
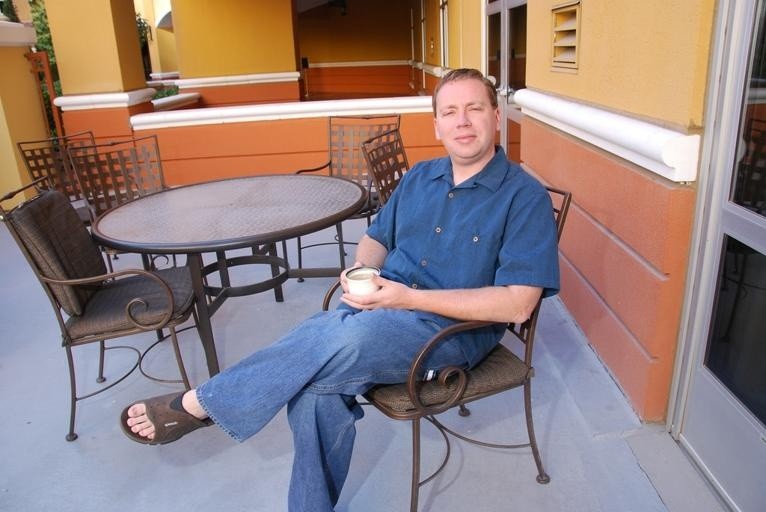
[{"xmin": 119, "ymin": 389, "xmax": 215, "ymax": 445}]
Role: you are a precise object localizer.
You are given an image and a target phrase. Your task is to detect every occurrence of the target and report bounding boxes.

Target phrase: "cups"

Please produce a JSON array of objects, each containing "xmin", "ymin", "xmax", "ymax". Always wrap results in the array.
[{"xmin": 345, "ymin": 265, "xmax": 381, "ymax": 297}]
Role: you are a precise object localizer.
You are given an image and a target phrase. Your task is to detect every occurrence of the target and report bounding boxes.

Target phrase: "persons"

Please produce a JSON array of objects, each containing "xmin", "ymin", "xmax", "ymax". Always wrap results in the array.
[{"xmin": 119, "ymin": 68, "xmax": 563, "ymax": 512}]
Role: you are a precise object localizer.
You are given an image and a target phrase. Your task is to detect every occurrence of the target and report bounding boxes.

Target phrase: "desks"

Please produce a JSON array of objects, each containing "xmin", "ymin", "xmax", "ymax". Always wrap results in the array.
[{"xmin": 91, "ymin": 173, "xmax": 364, "ymax": 377}]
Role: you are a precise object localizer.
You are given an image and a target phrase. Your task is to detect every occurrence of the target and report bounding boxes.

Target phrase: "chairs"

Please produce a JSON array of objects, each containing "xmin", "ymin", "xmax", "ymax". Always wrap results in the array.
[
  {"xmin": 16, "ymin": 131, "xmax": 119, "ymax": 261},
  {"xmin": 68, "ymin": 134, "xmax": 212, "ymax": 303},
  {"xmin": 324, "ymin": 186, "xmax": 571, "ymax": 512},
  {"xmin": 360, "ymin": 128, "xmax": 412, "ymax": 207},
  {"xmin": 0, "ymin": 175, "xmax": 198, "ymax": 443},
  {"xmin": 294, "ymin": 113, "xmax": 402, "ymax": 283}
]
